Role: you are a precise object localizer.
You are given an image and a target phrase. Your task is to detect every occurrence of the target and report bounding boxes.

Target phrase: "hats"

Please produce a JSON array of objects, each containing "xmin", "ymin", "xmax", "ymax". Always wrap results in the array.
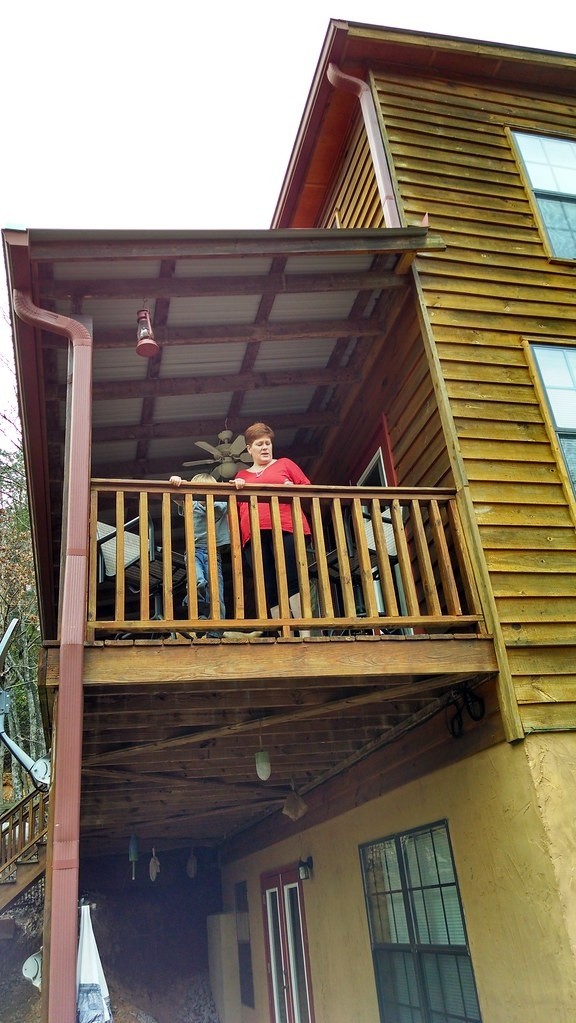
[{"xmin": 23, "ymin": 950, "xmax": 42, "ymax": 990}]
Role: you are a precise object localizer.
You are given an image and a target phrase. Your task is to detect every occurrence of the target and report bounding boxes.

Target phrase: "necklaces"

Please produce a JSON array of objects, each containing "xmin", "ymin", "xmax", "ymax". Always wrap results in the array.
[{"xmin": 252, "ymin": 463, "xmax": 268, "ymax": 478}]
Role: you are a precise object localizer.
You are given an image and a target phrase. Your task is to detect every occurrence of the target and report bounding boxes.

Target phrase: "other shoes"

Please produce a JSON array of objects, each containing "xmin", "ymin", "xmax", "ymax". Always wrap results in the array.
[
  {"xmin": 196, "ymin": 616, "xmax": 207, "ymax": 637},
  {"xmin": 208, "ymin": 621, "xmax": 224, "ymax": 638}
]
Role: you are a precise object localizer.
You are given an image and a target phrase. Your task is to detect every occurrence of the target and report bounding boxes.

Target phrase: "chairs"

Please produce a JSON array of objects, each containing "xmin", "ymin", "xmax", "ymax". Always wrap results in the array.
[
  {"xmin": 307, "ymin": 506, "xmax": 404, "ymax": 636},
  {"xmin": 96, "ymin": 510, "xmax": 194, "ymax": 640}
]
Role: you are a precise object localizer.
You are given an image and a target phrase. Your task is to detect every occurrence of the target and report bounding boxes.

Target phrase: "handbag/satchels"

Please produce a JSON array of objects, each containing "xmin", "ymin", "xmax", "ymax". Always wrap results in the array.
[{"xmin": 76, "ymin": 905, "xmax": 112, "ymax": 1023}]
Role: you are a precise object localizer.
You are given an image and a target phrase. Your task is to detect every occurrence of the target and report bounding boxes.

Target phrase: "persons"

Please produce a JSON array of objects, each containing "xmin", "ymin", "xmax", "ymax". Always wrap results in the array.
[
  {"xmin": 230, "ymin": 422, "xmax": 312, "ymax": 638},
  {"xmin": 169, "ymin": 473, "xmax": 245, "ymax": 639}
]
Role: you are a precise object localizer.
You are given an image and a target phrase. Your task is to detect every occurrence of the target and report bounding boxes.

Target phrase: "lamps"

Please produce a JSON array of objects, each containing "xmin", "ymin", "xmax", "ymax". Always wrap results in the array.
[
  {"xmin": 298, "ymin": 854, "xmax": 313, "ymax": 880},
  {"xmin": 135, "ymin": 297, "xmax": 159, "ymax": 357},
  {"xmin": 214, "ymin": 430, "xmax": 239, "ymax": 478}
]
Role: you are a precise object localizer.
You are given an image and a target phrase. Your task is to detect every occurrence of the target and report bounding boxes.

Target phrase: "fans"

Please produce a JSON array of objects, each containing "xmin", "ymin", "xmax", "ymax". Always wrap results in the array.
[{"xmin": 182, "ymin": 416, "xmax": 254, "ymax": 481}]
